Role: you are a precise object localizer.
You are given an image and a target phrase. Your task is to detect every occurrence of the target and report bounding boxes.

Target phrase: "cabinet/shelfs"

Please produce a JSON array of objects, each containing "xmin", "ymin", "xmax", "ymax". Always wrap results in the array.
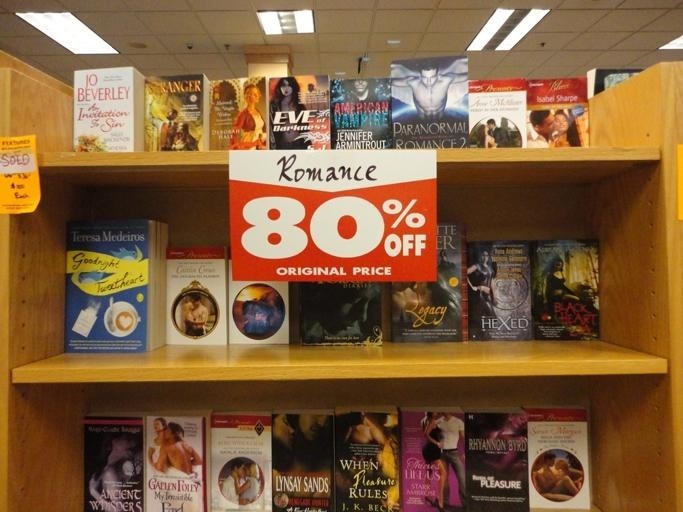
[{"xmin": 1, "ymin": 52, "xmax": 683, "ymax": 511}]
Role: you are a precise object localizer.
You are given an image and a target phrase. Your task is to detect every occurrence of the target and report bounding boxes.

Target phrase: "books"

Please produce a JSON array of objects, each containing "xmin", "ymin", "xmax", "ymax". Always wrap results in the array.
[
  {"xmin": 73, "ymin": 68, "xmax": 591, "ymax": 155},
  {"xmin": 65, "ymin": 218, "xmax": 601, "ymax": 355},
  {"xmin": 77, "ymin": 409, "xmax": 597, "ymax": 510}
]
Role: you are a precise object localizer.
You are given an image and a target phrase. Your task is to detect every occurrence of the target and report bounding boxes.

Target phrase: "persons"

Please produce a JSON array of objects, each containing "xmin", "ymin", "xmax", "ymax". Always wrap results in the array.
[
  {"xmin": 476, "ymin": 125, "xmax": 498, "ymax": 149},
  {"xmin": 272, "ymin": 414, "xmax": 296, "ymax": 473},
  {"xmin": 342, "ymin": 79, "xmax": 376, "ymax": 105},
  {"xmin": 420, "ymin": 412, "xmax": 467, "ymax": 508},
  {"xmin": 298, "ymin": 283, "xmax": 381, "ymax": 344},
  {"xmin": 220, "ymin": 460, "xmax": 244, "ymax": 503},
  {"xmin": 226, "ymin": 85, "xmax": 268, "ymax": 149},
  {"xmin": 389, "ymin": 60, "xmax": 468, "ymax": 125},
  {"xmin": 150, "ymin": 417, "xmax": 176, "ymax": 450},
  {"xmin": 535, "ymin": 452, "xmax": 578, "ymax": 500},
  {"xmin": 466, "ymin": 246, "xmax": 497, "ymax": 342},
  {"xmin": 553, "ymin": 459, "xmax": 583, "ymax": 489},
  {"xmin": 547, "ymin": 257, "xmax": 581, "ymax": 317},
  {"xmin": 420, "ymin": 412, "xmax": 447, "ymax": 507},
  {"xmin": 90, "ymin": 433, "xmax": 143, "ymax": 510},
  {"xmin": 486, "ymin": 119, "xmax": 511, "ymax": 148},
  {"xmin": 145, "ymin": 95, "xmax": 177, "ymax": 151},
  {"xmin": 298, "ymin": 414, "xmax": 332, "ymax": 443},
  {"xmin": 173, "ymin": 121, "xmax": 195, "ymax": 152},
  {"xmin": 185, "ymin": 293, "xmax": 209, "ymax": 335},
  {"xmin": 147, "ymin": 425, "xmax": 203, "ymax": 475},
  {"xmin": 528, "ymin": 110, "xmax": 557, "ymax": 148},
  {"xmin": 552, "ymin": 110, "xmax": 582, "ymax": 148},
  {"xmin": 235, "ymin": 463, "xmax": 262, "ymax": 505},
  {"xmin": 268, "ymin": 77, "xmax": 313, "ymax": 150}
]
